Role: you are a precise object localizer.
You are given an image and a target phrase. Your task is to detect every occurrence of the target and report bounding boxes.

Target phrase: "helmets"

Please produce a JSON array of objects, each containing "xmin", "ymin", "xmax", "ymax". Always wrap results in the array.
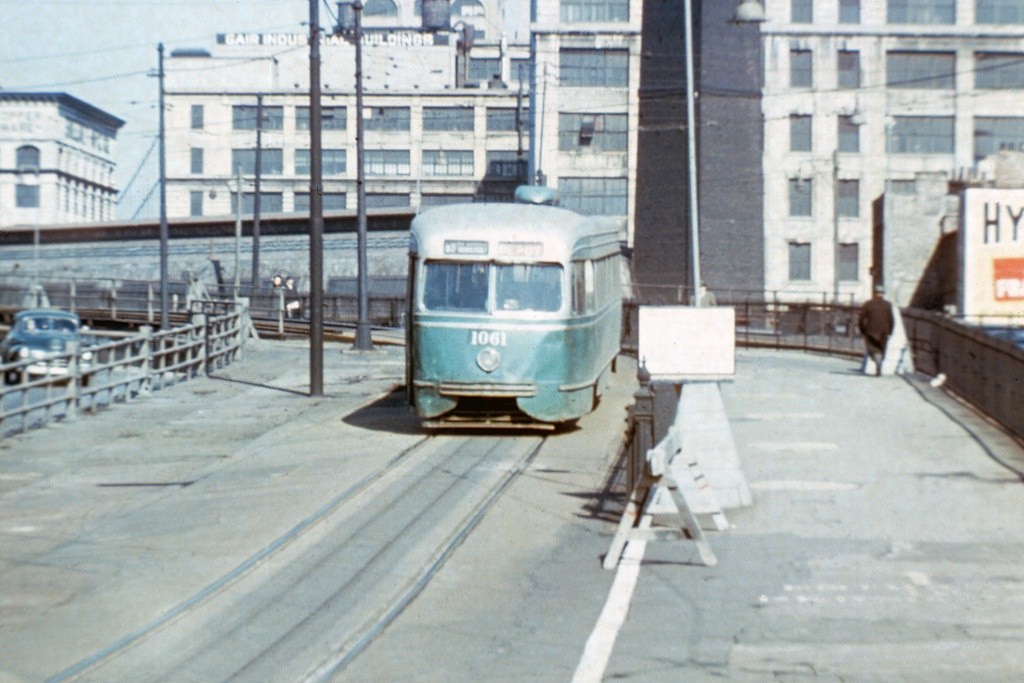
[{"xmin": 874, "ymin": 286, "xmax": 884, "ymax": 294}]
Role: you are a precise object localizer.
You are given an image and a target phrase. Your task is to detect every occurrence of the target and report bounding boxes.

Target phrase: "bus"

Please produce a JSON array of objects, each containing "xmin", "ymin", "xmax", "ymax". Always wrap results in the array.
[{"xmin": 404, "ymin": 185, "xmax": 623, "ymax": 435}]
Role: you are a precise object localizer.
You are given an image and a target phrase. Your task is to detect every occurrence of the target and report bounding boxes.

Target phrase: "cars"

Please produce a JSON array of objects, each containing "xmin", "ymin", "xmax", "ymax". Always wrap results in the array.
[{"xmin": 0, "ymin": 310, "xmax": 100, "ymax": 387}]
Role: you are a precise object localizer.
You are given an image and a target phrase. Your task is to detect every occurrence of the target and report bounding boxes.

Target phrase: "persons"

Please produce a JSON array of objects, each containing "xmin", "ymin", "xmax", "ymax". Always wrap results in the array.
[
  {"xmin": 273, "ymin": 276, "xmax": 305, "ymax": 319},
  {"xmin": 860, "ymin": 285, "xmax": 894, "ymax": 376},
  {"xmin": 699, "ymin": 284, "xmax": 716, "ymax": 307},
  {"xmin": 28, "ymin": 320, "xmax": 37, "ymax": 331}
]
